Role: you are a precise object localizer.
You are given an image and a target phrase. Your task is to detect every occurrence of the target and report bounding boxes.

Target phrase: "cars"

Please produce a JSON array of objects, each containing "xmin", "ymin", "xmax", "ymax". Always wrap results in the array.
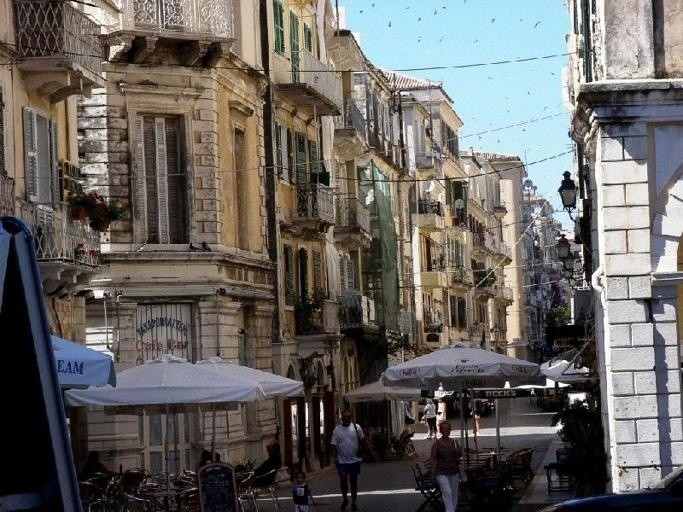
[{"xmin": 544, "ymin": 459, "xmax": 682, "ymax": 511}]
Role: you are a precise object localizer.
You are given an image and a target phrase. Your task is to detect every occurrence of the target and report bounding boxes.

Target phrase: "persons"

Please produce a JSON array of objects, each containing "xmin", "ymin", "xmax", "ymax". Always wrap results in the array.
[
  {"xmin": 198, "ymin": 450, "xmax": 211, "ymax": 467},
  {"xmin": 468, "ymin": 394, "xmax": 482, "ymax": 435},
  {"xmin": 430, "ymin": 421, "xmax": 463, "ymax": 512},
  {"xmin": 288, "ymin": 472, "xmax": 315, "ymax": 512},
  {"xmin": 79, "ymin": 451, "xmax": 104, "ymax": 482},
  {"xmin": 245, "ymin": 440, "xmax": 281, "ymax": 489},
  {"xmin": 436, "ymin": 398, "xmax": 447, "ymax": 437},
  {"xmin": 424, "ymin": 399, "xmax": 437, "ymax": 440},
  {"xmin": 330, "ymin": 409, "xmax": 365, "ymax": 512}
]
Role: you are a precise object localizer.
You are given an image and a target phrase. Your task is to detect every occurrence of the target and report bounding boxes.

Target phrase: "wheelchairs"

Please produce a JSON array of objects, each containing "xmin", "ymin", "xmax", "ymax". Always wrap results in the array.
[{"xmin": 392, "ymin": 430, "xmax": 418, "ymax": 460}]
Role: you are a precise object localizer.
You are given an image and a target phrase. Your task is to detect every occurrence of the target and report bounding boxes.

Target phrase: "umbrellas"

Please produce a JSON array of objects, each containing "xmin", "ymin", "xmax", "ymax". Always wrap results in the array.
[
  {"xmin": 50, "ymin": 333, "xmax": 117, "ymax": 409},
  {"xmin": 381, "ymin": 341, "xmax": 541, "ymax": 470},
  {"xmin": 63, "ymin": 352, "xmax": 266, "ymax": 476},
  {"xmin": 345, "ymin": 371, "xmax": 424, "ymax": 447},
  {"xmin": 193, "ymin": 355, "xmax": 305, "ymax": 463}
]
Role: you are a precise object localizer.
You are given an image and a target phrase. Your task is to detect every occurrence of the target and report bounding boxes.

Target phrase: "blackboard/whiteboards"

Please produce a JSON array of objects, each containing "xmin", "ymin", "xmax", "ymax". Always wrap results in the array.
[{"xmin": 198, "ymin": 463, "xmax": 239, "ymax": 512}]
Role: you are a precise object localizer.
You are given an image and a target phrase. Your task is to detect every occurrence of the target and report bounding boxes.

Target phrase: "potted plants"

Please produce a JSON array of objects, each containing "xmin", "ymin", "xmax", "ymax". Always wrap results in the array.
[{"xmin": 67, "ymin": 189, "xmax": 127, "ymax": 231}]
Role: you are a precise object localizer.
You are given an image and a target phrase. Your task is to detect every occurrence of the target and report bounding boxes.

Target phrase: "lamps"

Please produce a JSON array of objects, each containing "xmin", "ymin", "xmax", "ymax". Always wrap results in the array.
[
  {"xmin": 555, "ymin": 235, "xmax": 585, "ymax": 273},
  {"xmin": 558, "ymin": 171, "xmax": 582, "ymax": 224}
]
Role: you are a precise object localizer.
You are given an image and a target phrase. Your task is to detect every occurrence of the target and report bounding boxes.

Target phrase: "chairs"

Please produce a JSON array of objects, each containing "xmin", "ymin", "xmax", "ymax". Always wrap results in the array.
[
  {"xmin": 77, "ymin": 467, "xmax": 281, "ymax": 511},
  {"xmin": 411, "ymin": 447, "xmax": 574, "ymax": 512}
]
